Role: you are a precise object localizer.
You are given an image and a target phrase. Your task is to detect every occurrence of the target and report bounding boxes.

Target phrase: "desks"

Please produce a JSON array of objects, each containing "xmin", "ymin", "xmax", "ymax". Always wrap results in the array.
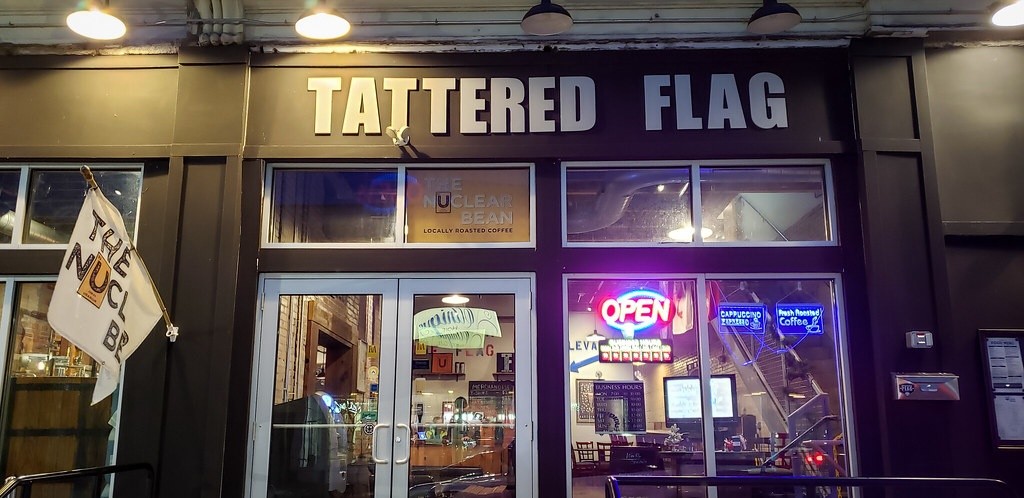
[
  {"xmin": 659, "ymin": 451, "xmax": 771, "ymax": 498},
  {"xmin": 611, "ymin": 445, "xmax": 665, "ymax": 475}
]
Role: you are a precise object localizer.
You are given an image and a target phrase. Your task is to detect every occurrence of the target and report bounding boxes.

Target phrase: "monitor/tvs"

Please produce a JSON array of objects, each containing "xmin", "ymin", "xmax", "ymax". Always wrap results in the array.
[{"xmin": 663, "ymin": 374, "xmax": 738, "ymax": 423}]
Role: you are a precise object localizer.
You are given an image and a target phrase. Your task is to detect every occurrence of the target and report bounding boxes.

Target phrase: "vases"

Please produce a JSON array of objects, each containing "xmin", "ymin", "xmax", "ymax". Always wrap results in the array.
[{"xmin": 672, "ymin": 445, "xmax": 675, "ymax": 452}]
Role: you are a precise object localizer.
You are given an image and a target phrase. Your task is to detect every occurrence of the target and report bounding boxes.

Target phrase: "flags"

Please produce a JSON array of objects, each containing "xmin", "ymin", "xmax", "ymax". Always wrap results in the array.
[{"xmin": 46, "ymin": 187, "xmax": 163, "ymax": 405}]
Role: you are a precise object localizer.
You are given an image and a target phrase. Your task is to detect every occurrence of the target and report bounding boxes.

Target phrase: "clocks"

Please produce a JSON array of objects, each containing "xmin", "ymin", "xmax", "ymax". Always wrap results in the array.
[{"xmin": 368, "ymin": 366, "xmax": 378, "ymax": 377}]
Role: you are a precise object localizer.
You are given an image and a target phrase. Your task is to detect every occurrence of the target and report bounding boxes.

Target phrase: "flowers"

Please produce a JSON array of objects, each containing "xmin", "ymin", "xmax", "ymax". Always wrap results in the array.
[{"xmin": 664, "ymin": 423, "xmax": 684, "ymax": 446}]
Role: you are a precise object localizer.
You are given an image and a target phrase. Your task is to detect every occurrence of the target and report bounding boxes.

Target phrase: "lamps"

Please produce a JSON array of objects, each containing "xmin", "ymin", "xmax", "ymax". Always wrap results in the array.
[
  {"xmin": 316, "ymin": 348, "xmax": 326, "ymax": 379},
  {"xmin": 586, "ymin": 312, "xmax": 606, "ymax": 342},
  {"xmin": 746, "ymin": 0, "xmax": 802, "ymax": 35},
  {"xmin": 386, "ymin": 125, "xmax": 411, "ymax": 146},
  {"xmin": 989, "ymin": 0, "xmax": 1024, "ymax": 26},
  {"xmin": 65, "ymin": 0, "xmax": 126, "ymax": 40},
  {"xmin": 294, "ymin": 0, "xmax": 351, "ymax": 40},
  {"xmin": 520, "ymin": 0, "xmax": 574, "ymax": 35}
]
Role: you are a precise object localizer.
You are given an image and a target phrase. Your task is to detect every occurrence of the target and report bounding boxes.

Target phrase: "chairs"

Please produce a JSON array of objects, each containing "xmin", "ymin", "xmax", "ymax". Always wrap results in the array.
[
  {"xmin": 605, "ymin": 476, "xmax": 621, "ymax": 498},
  {"xmin": 570, "ymin": 441, "xmax": 633, "ymax": 476}
]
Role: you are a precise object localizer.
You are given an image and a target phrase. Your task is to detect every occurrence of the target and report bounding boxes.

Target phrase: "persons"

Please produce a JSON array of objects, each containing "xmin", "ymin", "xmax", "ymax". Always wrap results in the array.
[
  {"xmin": 426, "ymin": 416, "xmax": 447, "ymax": 440},
  {"xmin": 448, "ymin": 397, "xmax": 480, "ymax": 445}
]
